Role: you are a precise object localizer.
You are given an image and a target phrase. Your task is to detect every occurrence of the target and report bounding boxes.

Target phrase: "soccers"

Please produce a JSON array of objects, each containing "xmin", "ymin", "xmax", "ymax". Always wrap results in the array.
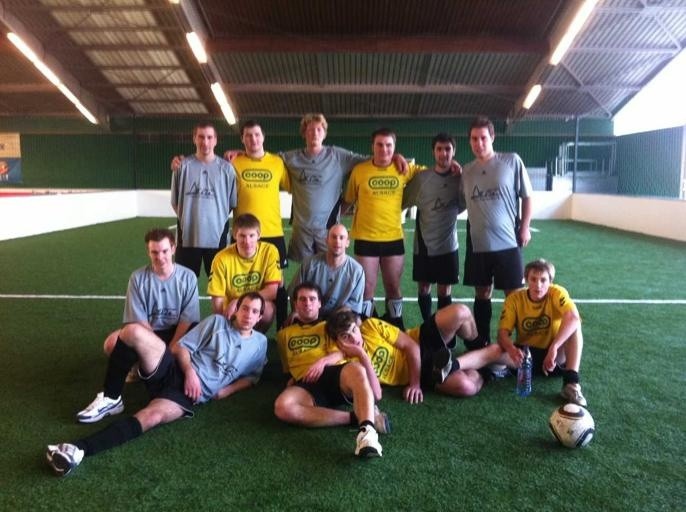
[{"xmin": 548, "ymin": 404, "xmax": 595, "ymax": 450}]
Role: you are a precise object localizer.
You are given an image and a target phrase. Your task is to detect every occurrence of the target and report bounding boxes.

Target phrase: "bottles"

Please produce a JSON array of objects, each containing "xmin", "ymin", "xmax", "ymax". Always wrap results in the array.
[{"xmin": 516, "ymin": 344, "xmax": 532, "ymax": 397}]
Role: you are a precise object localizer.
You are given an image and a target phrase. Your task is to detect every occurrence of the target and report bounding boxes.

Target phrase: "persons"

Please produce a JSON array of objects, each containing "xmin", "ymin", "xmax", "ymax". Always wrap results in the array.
[
  {"xmin": 170, "ymin": 121, "xmax": 238, "ymax": 279},
  {"xmin": 460, "ymin": 118, "xmax": 533, "ymax": 341},
  {"xmin": 206, "ymin": 213, "xmax": 283, "ymax": 334},
  {"xmin": 341, "ymin": 123, "xmax": 463, "ymax": 332},
  {"xmin": 45, "ymin": 290, "xmax": 268, "ymax": 479},
  {"xmin": 432, "ymin": 259, "xmax": 587, "ymax": 407},
  {"xmin": 288, "ymin": 223, "xmax": 364, "ymax": 319},
  {"xmin": 401, "ymin": 132, "xmax": 466, "ymax": 350},
  {"xmin": 274, "ymin": 282, "xmax": 390, "ymax": 456},
  {"xmin": 103, "ymin": 228, "xmax": 200, "ymax": 383},
  {"xmin": 325, "ymin": 303, "xmax": 507, "ymax": 404},
  {"xmin": 169, "ymin": 118, "xmax": 293, "ymax": 285},
  {"xmin": 224, "ymin": 112, "xmax": 409, "ymax": 260}
]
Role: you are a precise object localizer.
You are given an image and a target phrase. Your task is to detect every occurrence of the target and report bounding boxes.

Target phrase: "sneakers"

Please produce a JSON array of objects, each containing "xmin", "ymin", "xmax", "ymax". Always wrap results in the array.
[
  {"xmin": 125, "ymin": 361, "xmax": 141, "ymax": 384},
  {"xmin": 75, "ymin": 390, "xmax": 127, "ymax": 422},
  {"xmin": 355, "ymin": 424, "xmax": 384, "ymax": 457},
  {"xmin": 562, "ymin": 382, "xmax": 589, "ymax": 407},
  {"xmin": 430, "ymin": 347, "xmax": 454, "ymax": 384},
  {"xmin": 372, "ymin": 405, "xmax": 392, "ymax": 435},
  {"xmin": 44, "ymin": 443, "xmax": 86, "ymax": 477},
  {"xmin": 486, "ymin": 363, "xmax": 515, "ymax": 379}
]
